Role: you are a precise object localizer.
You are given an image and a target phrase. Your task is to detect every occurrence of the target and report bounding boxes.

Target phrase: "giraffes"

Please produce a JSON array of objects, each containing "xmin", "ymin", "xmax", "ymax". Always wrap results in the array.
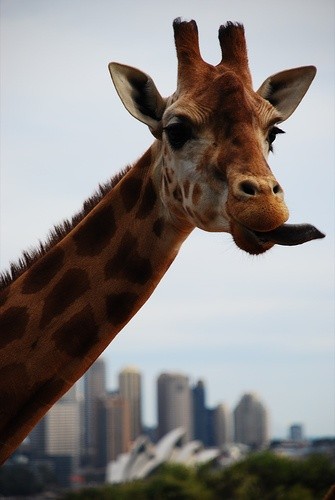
[{"xmin": 0, "ymin": 18, "xmax": 326, "ymax": 470}]
